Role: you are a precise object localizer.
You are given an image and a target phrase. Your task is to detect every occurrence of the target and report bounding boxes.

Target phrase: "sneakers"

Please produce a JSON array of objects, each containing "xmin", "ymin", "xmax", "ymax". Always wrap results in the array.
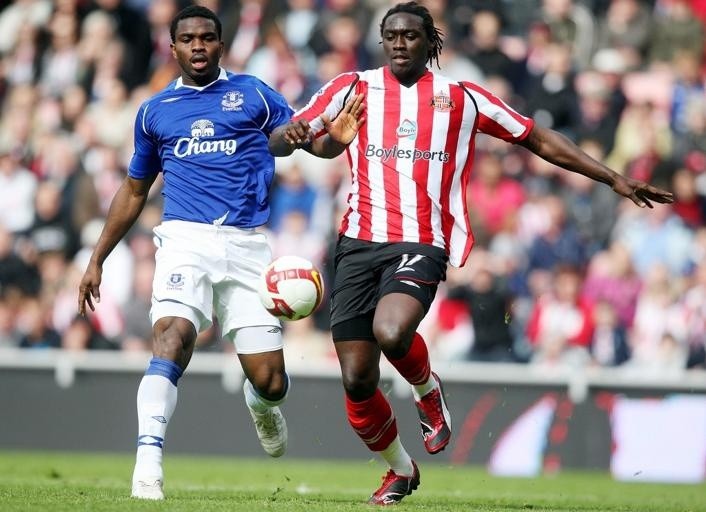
[
  {"xmin": 131, "ymin": 479, "xmax": 164, "ymax": 501},
  {"xmin": 368, "ymin": 458, "xmax": 420, "ymax": 505},
  {"xmin": 243, "ymin": 378, "xmax": 288, "ymax": 457},
  {"xmin": 414, "ymin": 372, "xmax": 452, "ymax": 454}
]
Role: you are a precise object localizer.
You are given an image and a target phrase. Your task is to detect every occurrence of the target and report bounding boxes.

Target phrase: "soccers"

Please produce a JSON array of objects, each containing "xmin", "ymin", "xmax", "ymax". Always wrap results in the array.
[{"xmin": 259, "ymin": 255, "xmax": 324, "ymax": 321}]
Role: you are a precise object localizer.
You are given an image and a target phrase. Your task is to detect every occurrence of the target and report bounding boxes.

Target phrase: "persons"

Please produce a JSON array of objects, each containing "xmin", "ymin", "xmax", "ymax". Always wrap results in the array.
[
  {"xmin": 73, "ymin": 7, "xmax": 366, "ymax": 500},
  {"xmin": 272, "ymin": 3, "xmax": 676, "ymax": 505},
  {"xmin": 2, "ymin": 1, "xmax": 706, "ymax": 387}
]
